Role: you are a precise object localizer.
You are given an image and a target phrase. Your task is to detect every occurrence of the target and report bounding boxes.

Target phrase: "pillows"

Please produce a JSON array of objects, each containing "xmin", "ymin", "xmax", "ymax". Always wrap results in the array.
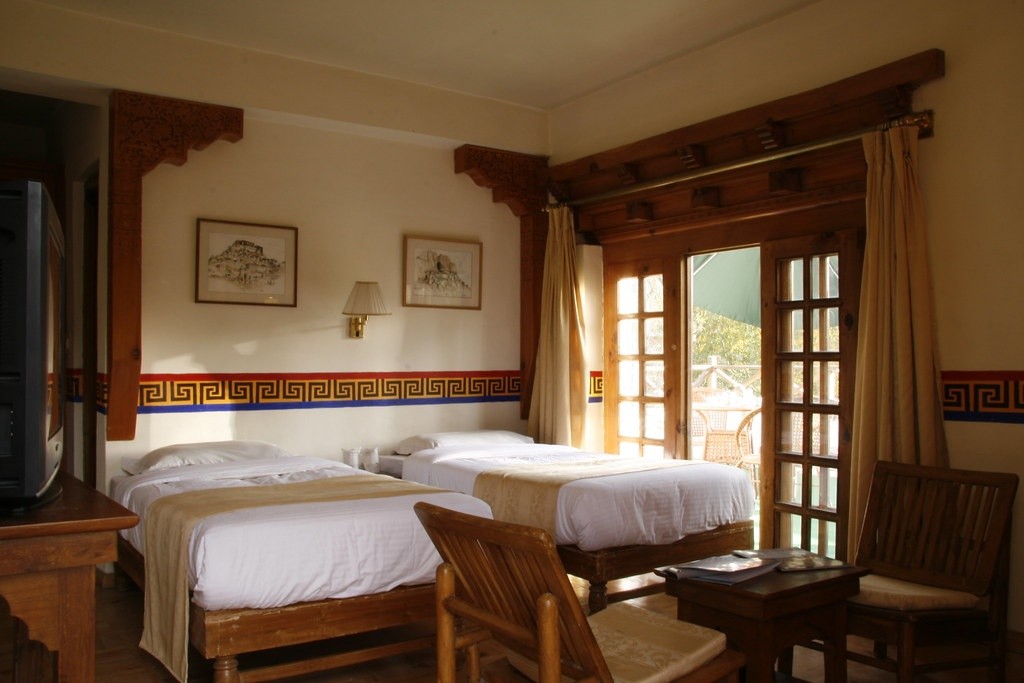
[
  {"xmin": 392, "ymin": 430, "xmax": 534, "ymax": 455},
  {"xmin": 122, "ymin": 442, "xmax": 296, "ymax": 474}
]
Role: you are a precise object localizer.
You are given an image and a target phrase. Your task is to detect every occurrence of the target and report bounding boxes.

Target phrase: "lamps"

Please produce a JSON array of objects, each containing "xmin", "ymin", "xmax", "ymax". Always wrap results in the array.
[{"xmin": 342, "ymin": 281, "xmax": 392, "ymax": 339}]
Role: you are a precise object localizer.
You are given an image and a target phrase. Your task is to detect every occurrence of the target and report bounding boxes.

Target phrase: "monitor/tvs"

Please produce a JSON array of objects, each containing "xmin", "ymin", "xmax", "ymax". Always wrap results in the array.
[{"xmin": 0, "ymin": 182, "xmax": 65, "ymax": 512}]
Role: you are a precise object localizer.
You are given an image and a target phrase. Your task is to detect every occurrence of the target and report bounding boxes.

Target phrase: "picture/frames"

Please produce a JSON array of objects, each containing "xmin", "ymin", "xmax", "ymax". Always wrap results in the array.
[
  {"xmin": 195, "ymin": 217, "xmax": 298, "ymax": 308},
  {"xmin": 402, "ymin": 233, "xmax": 483, "ymax": 311}
]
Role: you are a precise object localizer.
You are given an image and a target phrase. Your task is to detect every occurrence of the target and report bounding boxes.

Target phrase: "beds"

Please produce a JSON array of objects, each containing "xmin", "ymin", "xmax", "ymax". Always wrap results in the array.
[
  {"xmin": 362, "ymin": 429, "xmax": 754, "ymax": 617},
  {"xmin": 110, "ymin": 441, "xmax": 493, "ymax": 683}
]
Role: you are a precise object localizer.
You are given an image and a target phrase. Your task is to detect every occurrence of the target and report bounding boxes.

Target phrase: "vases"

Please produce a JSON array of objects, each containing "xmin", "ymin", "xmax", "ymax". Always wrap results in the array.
[
  {"xmin": 341, "ymin": 446, "xmax": 361, "ymax": 469},
  {"xmin": 362, "ymin": 444, "xmax": 380, "ymax": 473}
]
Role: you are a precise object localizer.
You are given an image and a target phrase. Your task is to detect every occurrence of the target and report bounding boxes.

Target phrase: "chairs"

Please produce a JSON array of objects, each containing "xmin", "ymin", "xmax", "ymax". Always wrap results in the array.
[
  {"xmin": 778, "ymin": 460, "xmax": 1019, "ymax": 683},
  {"xmin": 413, "ymin": 501, "xmax": 748, "ymax": 683}
]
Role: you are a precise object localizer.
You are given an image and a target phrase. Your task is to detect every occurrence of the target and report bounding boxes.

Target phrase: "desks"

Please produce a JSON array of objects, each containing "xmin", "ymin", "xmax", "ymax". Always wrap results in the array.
[
  {"xmin": 0, "ymin": 470, "xmax": 141, "ymax": 683},
  {"xmin": 653, "ymin": 548, "xmax": 871, "ymax": 683}
]
